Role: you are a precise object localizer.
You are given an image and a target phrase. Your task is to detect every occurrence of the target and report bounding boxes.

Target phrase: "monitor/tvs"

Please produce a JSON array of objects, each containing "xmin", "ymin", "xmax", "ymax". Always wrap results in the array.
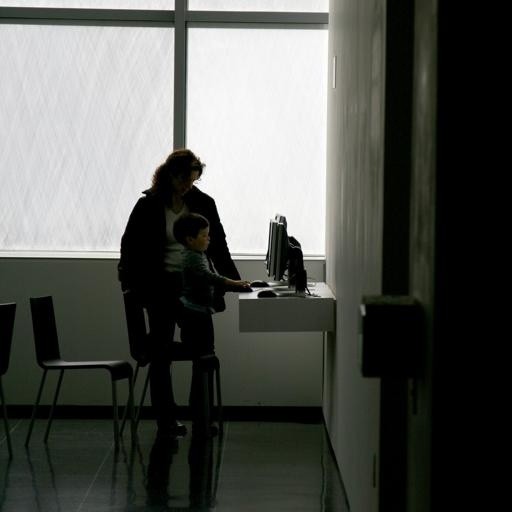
[{"xmin": 265, "ymin": 215, "xmax": 307, "ymax": 297}]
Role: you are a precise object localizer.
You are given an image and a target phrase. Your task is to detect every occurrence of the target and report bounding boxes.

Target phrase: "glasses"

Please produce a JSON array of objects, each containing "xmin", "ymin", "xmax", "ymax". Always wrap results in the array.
[{"xmin": 180, "ymin": 175, "xmax": 199, "ymax": 184}]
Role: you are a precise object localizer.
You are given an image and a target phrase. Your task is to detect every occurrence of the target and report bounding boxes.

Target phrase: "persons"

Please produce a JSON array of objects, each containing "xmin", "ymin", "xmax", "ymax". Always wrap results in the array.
[{"xmin": 118, "ymin": 149, "xmax": 252, "ymax": 436}]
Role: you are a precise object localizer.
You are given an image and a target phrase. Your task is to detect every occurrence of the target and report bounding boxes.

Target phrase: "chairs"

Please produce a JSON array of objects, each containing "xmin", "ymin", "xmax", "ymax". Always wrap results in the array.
[{"xmin": 0, "ymin": 291, "xmax": 224, "ymax": 458}]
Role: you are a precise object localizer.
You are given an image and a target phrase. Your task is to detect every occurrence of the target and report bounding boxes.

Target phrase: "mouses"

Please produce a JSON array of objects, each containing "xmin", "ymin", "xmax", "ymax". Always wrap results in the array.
[
  {"xmin": 251, "ymin": 282, "xmax": 268, "ymax": 287},
  {"xmin": 232, "ymin": 286, "xmax": 252, "ymax": 292},
  {"xmin": 258, "ymin": 291, "xmax": 276, "ymax": 298}
]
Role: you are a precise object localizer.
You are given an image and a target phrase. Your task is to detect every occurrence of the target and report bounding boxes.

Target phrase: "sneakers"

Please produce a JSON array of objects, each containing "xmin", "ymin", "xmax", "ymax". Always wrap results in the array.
[
  {"xmin": 191, "ymin": 421, "xmax": 220, "ymax": 437},
  {"xmin": 157, "ymin": 419, "xmax": 188, "ymax": 436}
]
